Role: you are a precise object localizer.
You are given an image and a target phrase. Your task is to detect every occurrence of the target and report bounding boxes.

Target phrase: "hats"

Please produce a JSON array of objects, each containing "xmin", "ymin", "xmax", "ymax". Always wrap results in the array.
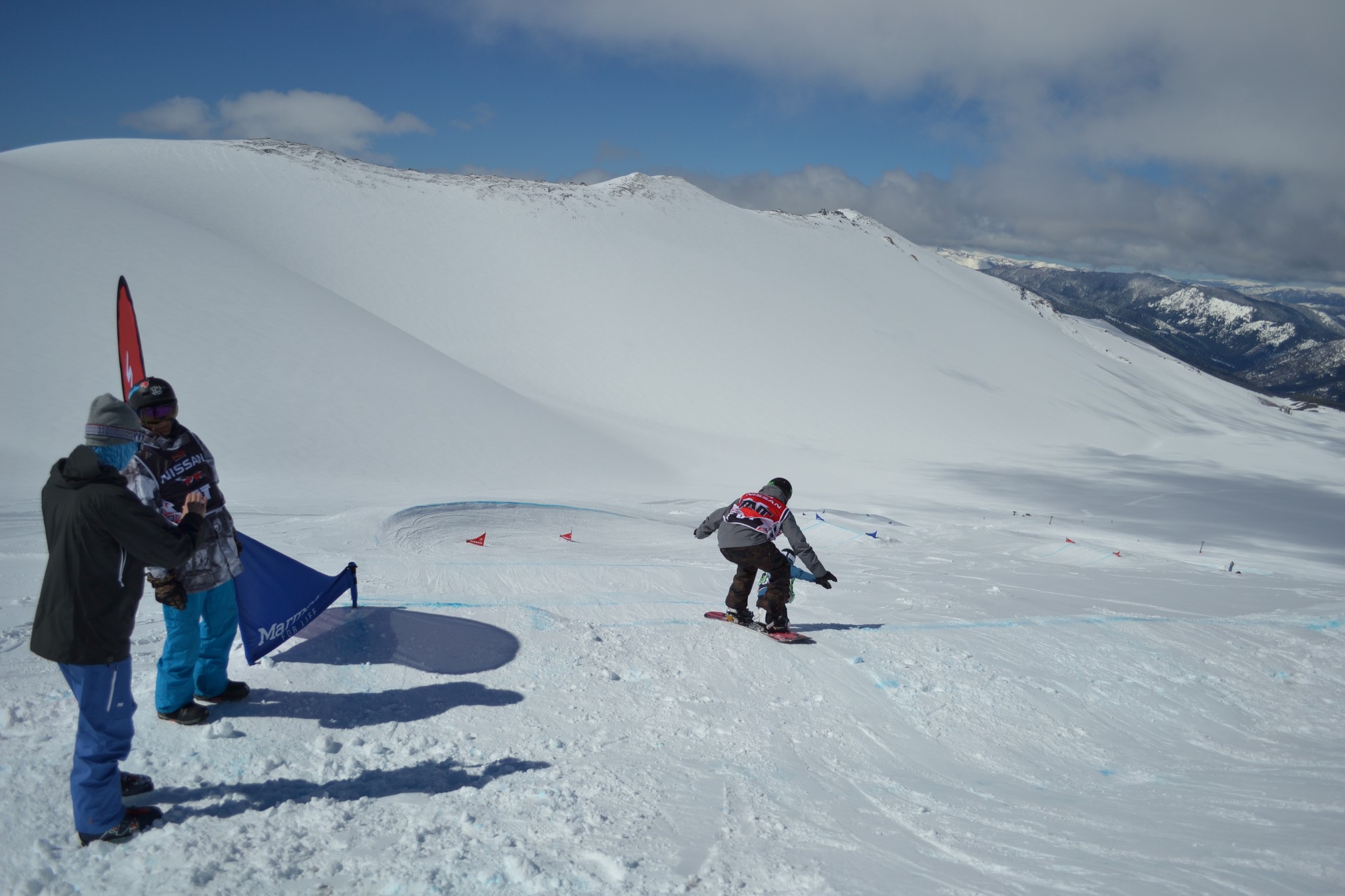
[{"xmin": 84, "ymin": 393, "xmax": 143, "ymax": 447}]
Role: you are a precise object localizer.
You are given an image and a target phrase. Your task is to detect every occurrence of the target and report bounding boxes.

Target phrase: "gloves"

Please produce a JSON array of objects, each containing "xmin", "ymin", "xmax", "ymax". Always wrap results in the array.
[
  {"xmin": 814, "ymin": 578, "xmax": 832, "ymax": 589},
  {"xmin": 816, "ymin": 571, "xmax": 837, "ymax": 582},
  {"xmin": 693, "ymin": 528, "xmax": 698, "ymax": 537},
  {"xmin": 144, "ymin": 568, "xmax": 188, "ymax": 611},
  {"xmin": 233, "ymin": 531, "xmax": 244, "ymax": 558}
]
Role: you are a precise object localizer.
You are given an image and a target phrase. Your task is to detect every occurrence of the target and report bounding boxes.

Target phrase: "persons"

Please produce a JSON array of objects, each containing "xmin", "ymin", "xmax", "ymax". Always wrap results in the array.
[
  {"xmin": 30, "ymin": 394, "xmax": 210, "ymax": 844},
  {"xmin": 694, "ymin": 478, "xmax": 837, "ymax": 630},
  {"xmin": 756, "ymin": 548, "xmax": 832, "ymax": 627},
  {"xmin": 119, "ymin": 378, "xmax": 250, "ymax": 725}
]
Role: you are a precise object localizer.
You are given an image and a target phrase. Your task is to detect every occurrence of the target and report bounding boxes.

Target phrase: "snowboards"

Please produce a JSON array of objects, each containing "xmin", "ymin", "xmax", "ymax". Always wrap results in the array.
[{"xmin": 704, "ymin": 612, "xmax": 813, "ymax": 643}]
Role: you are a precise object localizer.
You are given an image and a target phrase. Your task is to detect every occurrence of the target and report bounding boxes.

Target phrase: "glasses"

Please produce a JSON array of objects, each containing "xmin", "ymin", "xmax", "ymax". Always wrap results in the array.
[{"xmin": 138, "ymin": 400, "xmax": 179, "ymax": 425}]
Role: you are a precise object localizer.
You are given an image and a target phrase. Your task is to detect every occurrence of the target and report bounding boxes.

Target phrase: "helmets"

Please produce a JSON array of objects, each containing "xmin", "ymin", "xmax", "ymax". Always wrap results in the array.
[
  {"xmin": 128, "ymin": 376, "xmax": 178, "ymax": 415},
  {"xmin": 780, "ymin": 548, "xmax": 796, "ymax": 564},
  {"xmin": 766, "ymin": 477, "xmax": 792, "ymax": 500}
]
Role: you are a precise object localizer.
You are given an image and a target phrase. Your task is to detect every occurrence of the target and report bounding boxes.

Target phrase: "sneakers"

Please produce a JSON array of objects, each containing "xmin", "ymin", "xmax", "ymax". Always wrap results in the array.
[
  {"xmin": 118, "ymin": 770, "xmax": 154, "ymax": 797},
  {"xmin": 194, "ymin": 679, "xmax": 251, "ymax": 702},
  {"xmin": 78, "ymin": 804, "xmax": 164, "ymax": 848},
  {"xmin": 725, "ymin": 607, "xmax": 754, "ymax": 624},
  {"xmin": 752, "ymin": 607, "xmax": 790, "ymax": 634},
  {"xmin": 157, "ymin": 701, "xmax": 210, "ymax": 725}
]
